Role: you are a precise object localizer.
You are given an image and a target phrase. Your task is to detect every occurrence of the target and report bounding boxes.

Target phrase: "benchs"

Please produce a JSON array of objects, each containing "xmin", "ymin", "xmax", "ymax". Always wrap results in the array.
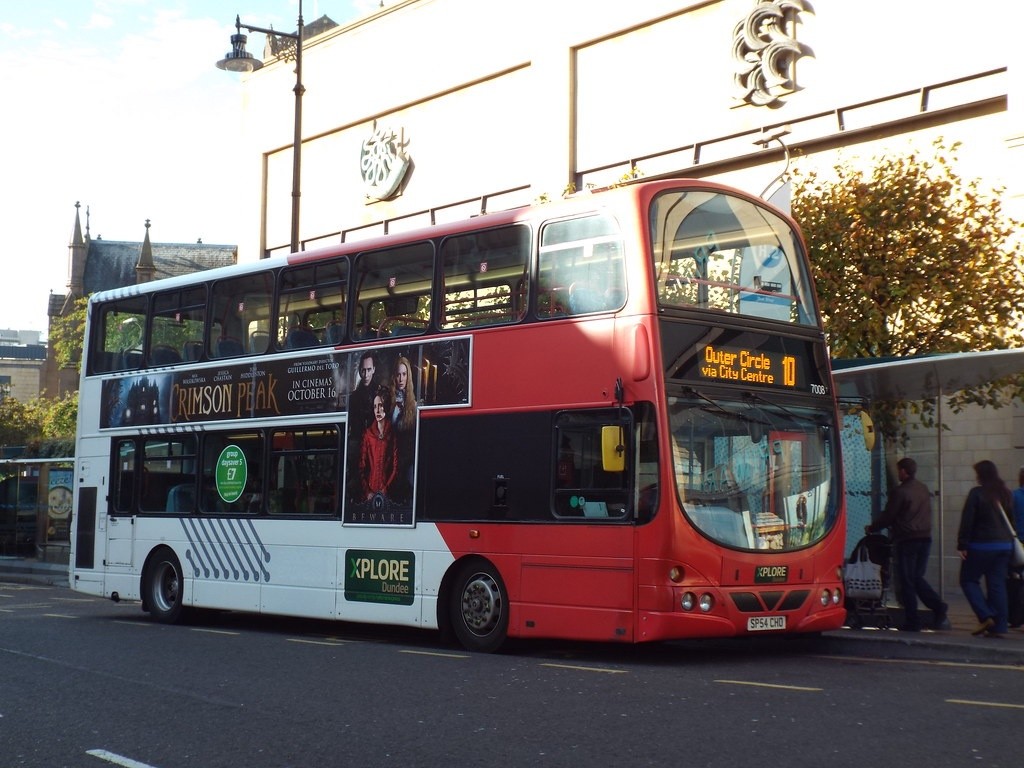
[{"xmin": 37, "ymin": 542, "xmax": 70, "ymax": 560}]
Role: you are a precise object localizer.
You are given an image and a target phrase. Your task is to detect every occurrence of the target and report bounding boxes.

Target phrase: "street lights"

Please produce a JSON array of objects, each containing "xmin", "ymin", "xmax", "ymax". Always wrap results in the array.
[{"xmin": 213, "ymin": 1, "xmax": 316, "ymax": 253}]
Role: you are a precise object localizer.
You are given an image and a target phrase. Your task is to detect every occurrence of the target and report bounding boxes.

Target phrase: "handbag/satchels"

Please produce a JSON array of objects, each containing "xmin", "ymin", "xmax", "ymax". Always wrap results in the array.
[
  {"xmin": 845, "ymin": 545, "xmax": 883, "ymax": 599},
  {"xmin": 1013, "ymin": 536, "xmax": 1024, "ymax": 567}
]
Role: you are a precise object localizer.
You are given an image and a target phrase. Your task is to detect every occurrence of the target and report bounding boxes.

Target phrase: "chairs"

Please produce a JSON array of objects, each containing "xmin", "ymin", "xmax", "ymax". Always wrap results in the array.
[
  {"xmin": 182, "ymin": 340, "xmax": 205, "ymax": 361},
  {"xmin": 166, "ymin": 484, "xmax": 195, "ymax": 515},
  {"xmin": 150, "ymin": 344, "xmax": 181, "ymax": 367},
  {"xmin": 123, "ymin": 348, "xmax": 143, "ymax": 368},
  {"xmin": 215, "ymin": 336, "xmax": 243, "ymax": 358},
  {"xmin": 249, "ymin": 331, "xmax": 270, "ymax": 353},
  {"xmin": 360, "ymin": 325, "xmax": 389, "ymax": 341},
  {"xmin": 286, "ymin": 325, "xmax": 321, "ymax": 349},
  {"xmin": 325, "ymin": 320, "xmax": 346, "ymax": 345}
]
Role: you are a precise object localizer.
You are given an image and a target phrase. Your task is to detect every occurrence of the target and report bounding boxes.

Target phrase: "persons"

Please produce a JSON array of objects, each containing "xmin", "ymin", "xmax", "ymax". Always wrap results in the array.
[
  {"xmin": 796, "ymin": 495, "xmax": 807, "ymax": 525},
  {"xmin": 347, "ymin": 353, "xmax": 391, "ymax": 441},
  {"xmin": 956, "ymin": 460, "xmax": 1017, "ymax": 637},
  {"xmin": 391, "ymin": 357, "xmax": 418, "ymax": 465},
  {"xmin": 358, "ymin": 385, "xmax": 401, "ymax": 500},
  {"xmin": 864, "ymin": 458, "xmax": 948, "ymax": 632},
  {"xmin": 1006, "ymin": 465, "xmax": 1024, "ymax": 631}
]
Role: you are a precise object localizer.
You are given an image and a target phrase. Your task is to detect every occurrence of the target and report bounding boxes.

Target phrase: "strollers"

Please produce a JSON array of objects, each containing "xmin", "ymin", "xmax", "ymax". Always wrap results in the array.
[{"xmin": 846, "ymin": 526, "xmax": 896, "ymax": 630}]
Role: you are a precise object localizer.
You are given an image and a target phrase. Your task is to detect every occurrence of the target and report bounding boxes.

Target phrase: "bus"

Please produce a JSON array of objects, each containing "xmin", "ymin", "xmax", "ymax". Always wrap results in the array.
[{"xmin": 67, "ymin": 180, "xmax": 846, "ymax": 652}]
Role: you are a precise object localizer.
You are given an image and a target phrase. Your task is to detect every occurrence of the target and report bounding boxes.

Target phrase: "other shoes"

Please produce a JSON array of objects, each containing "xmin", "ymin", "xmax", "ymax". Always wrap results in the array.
[
  {"xmin": 1007, "ymin": 622, "xmax": 1021, "ymax": 628},
  {"xmin": 934, "ymin": 601, "xmax": 948, "ymax": 626},
  {"xmin": 970, "ymin": 618, "xmax": 995, "ymax": 635},
  {"xmin": 984, "ymin": 631, "xmax": 1003, "ymax": 638},
  {"xmin": 897, "ymin": 621, "xmax": 922, "ymax": 632}
]
